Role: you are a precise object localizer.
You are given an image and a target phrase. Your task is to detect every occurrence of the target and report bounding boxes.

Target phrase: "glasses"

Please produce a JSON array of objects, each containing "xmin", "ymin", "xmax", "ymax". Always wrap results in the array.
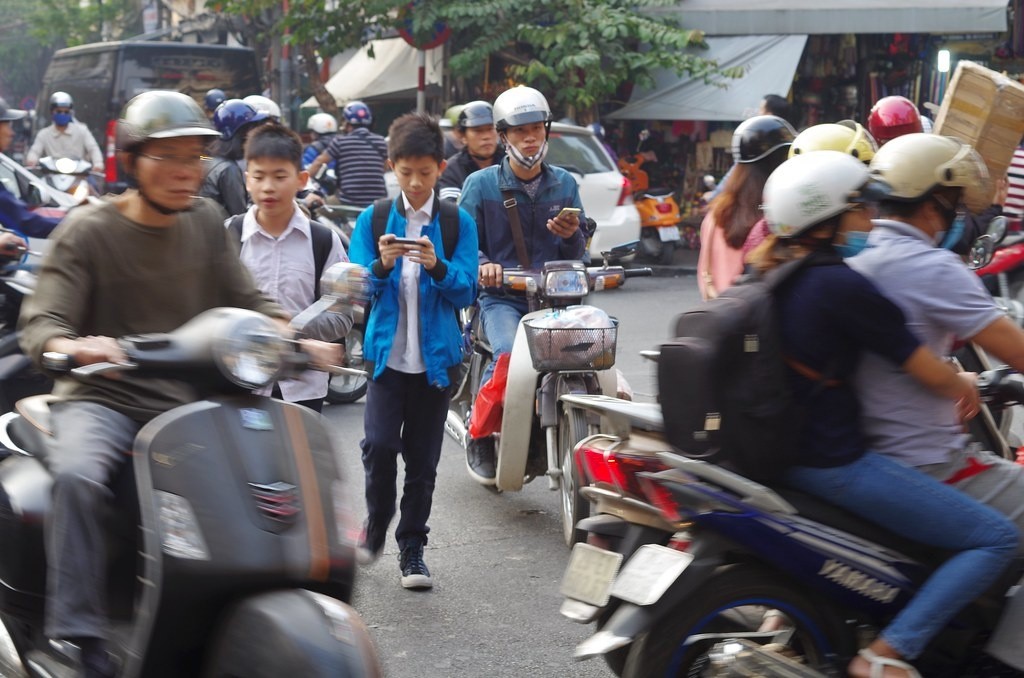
[
  {"xmin": 54, "ymin": 110, "xmax": 69, "ymax": 113},
  {"xmin": 134, "ymin": 152, "xmax": 213, "ymax": 171}
]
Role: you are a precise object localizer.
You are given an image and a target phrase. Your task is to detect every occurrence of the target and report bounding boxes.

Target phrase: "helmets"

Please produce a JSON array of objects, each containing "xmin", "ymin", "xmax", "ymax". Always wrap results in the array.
[
  {"xmin": 205, "ymin": 89, "xmax": 224, "ymax": 109},
  {"xmin": 869, "ymin": 133, "xmax": 973, "ymax": 203},
  {"xmin": 761, "ymin": 150, "xmax": 892, "ymax": 238},
  {"xmin": 459, "ymin": 100, "xmax": 494, "ymax": 128},
  {"xmin": 343, "ymin": 101, "xmax": 372, "ymax": 126},
  {"xmin": 445, "ymin": 104, "xmax": 463, "ymax": 128},
  {"xmin": 869, "ymin": 95, "xmax": 922, "ymax": 142},
  {"xmin": 732, "ymin": 116, "xmax": 797, "ymax": 163},
  {"xmin": 789, "ymin": 123, "xmax": 873, "ymax": 163},
  {"xmin": 214, "ymin": 99, "xmax": 267, "ymax": 141},
  {"xmin": 493, "ymin": 85, "xmax": 552, "ymax": 130},
  {"xmin": 242, "ymin": 95, "xmax": 281, "ymax": 123},
  {"xmin": 49, "ymin": 92, "xmax": 73, "ymax": 108},
  {"xmin": 307, "ymin": 113, "xmax": 337, "ymax": 134},
  {"xmin": 115, "ymin": 91, "xmax": 224, "ymax": 148}
]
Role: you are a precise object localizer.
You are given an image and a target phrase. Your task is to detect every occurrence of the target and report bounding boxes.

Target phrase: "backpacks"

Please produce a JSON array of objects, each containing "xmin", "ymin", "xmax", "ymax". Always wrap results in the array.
[{"xmin": 658, "ymin": 253, "xmax": 842, "ymax": 469}]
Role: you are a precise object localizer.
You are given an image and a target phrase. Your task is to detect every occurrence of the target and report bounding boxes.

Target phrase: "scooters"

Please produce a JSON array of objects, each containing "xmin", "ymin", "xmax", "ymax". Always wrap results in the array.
[
  {"xmin": 36, "ymin": 153, "xmax": 99, "ymax": 206},
  {"xmin": 611, "ymin": 128, "xmax": 684, "ymax": 265},
  {"xmin": 1, "ymin": 263, "xmax": 394, "ymax": 678},
  {"xmin": 283, "ymin": 189, "xmax": 368, "ymax": 406},
  {"xmin": 442, "ymin": 241, "xmax": 653, "ymax": 563},
  {"xmin": 561, "ymin": 215, "xmax": 1024, "ymax": 678}
]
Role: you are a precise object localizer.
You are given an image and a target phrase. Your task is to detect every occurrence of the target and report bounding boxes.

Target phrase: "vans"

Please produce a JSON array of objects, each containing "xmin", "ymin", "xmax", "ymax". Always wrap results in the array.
[{"xmin": 26, "ymin": 40, "xmax": 262, "ymax": 196}]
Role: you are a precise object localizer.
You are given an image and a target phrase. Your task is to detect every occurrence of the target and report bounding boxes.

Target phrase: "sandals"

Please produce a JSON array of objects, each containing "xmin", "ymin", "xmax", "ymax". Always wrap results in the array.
[{"xmin": 857, "ymin": 647, "xmax": 921, "ymax": 677}]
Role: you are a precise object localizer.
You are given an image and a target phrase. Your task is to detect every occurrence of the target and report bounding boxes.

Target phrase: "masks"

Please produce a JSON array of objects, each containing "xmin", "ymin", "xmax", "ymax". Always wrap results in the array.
[
  {"xmin": 501, "ymin": 135, "xmax": 549, "ymax": 171},
  {"xmin": 52, "ymin": 115, "xmax": 71, "ymax": 125}
]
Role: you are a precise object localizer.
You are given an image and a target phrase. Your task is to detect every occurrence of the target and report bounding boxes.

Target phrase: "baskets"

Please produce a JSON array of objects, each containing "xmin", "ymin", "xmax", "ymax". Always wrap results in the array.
[{"xmin": 522, "ymin": 313, "xmax": 618, "ymax": 370}]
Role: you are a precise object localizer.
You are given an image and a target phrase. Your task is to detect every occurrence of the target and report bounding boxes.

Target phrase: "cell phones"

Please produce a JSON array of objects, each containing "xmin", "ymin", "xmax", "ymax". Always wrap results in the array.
[
  {"xmin": 557, "ymin": 208, "xmax": 581, "ymax": 221},
  {"xmin": 388, "ymin": 237, "xmax": 426, "ymax": 249}
]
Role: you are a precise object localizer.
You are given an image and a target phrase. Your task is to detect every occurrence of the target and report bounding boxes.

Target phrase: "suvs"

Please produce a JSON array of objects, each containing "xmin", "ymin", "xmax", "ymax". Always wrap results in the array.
[{"xmin": 378, "ymin": 118, "xmax": 643, "ymax": 264}]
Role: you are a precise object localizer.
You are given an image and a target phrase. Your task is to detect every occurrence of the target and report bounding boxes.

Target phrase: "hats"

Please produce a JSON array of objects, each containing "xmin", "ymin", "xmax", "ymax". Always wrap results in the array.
[{"xmin": 0, "ymin": 98, "xmax": 27, "ymax": 122}]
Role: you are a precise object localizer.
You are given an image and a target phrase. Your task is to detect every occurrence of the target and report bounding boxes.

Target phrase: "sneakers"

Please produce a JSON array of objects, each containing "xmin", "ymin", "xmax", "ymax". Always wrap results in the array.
[
  {"xmin": 395, "ymin": 544, "xmax": 431, "ymax": 588},
  {"xmin": 465, "ymin": 432, "xmax": 497, "ymax": 485},
  {"xmin": 356, "ymin": 515, "xmax": 388, "ymax": 567}
]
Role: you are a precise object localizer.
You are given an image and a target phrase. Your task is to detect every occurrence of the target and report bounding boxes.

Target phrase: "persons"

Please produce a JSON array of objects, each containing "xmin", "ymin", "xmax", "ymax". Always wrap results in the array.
[
  {"xmin": 349, "ymin": 109, "xmax": 479, "ymax": 590},
  {"xmin": 433, "ymin": 84, "xmax": 589, "ymax": 486},
  {"xmin": 0, "ymin": 92, "xmax": 104, "ymax": 334},
  {"xmin": 18, "ymin": 91, "xmax": 345, "ymax": 678},
  {"xmin": 192, "ymin": 89, "xmax": 393, "ymax": 419},
  {"xmin": 698, "ymin": 94, "xmax": 1024, "ymax": 678}
]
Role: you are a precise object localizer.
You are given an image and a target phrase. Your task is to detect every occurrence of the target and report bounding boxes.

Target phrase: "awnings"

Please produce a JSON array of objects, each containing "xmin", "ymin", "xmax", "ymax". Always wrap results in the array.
[
  {"xmin": 600, "ymin": 34, "xmax": 807, "ymax": 120},
  {"xmin": 299, "ymin": 37, "xmax": 444, "ymax": 108}
]
